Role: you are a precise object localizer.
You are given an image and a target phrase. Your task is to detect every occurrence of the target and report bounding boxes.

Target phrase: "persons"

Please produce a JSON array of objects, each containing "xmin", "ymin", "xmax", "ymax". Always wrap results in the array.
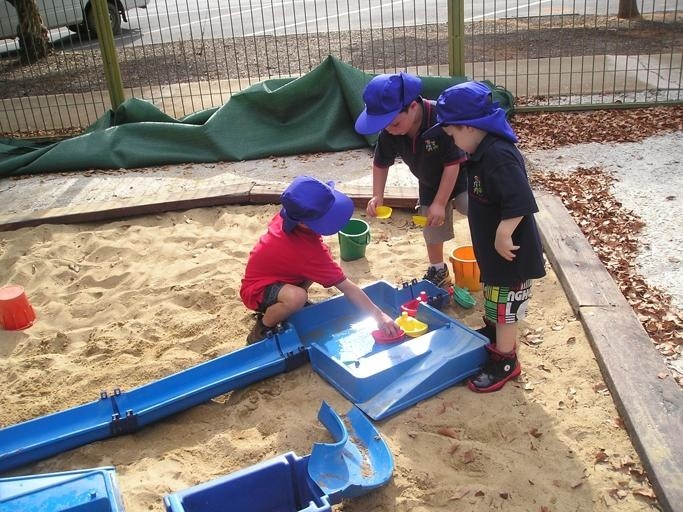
[
  {"xmin": 422, "ymin": 79, "xmax": 547, "ymax": 393},
  {"xmin": 238, "ymin": 174, "xmax": 402, "ymax": 345},
  {"xmin": 355, "ymin": 72, "xmax": 470, "ymax": 288}
]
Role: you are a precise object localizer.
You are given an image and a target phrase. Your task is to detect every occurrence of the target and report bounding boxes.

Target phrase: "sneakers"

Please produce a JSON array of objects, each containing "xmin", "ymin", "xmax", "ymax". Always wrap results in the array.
[{"xmin": 422, "ymin": 263, "xmax": 450, "ymax": 288}]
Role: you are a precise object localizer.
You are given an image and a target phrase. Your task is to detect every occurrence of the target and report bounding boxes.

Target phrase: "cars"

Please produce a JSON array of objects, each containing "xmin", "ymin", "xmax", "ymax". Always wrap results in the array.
[{"xmin": 0, "ymin": 0, "xmax": 150, "ymax": 39}]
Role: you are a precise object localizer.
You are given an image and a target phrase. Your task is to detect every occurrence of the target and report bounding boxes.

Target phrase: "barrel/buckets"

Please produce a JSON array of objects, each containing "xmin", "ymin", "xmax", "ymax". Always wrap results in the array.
[
  {"xmin": 450, "ymin": 244, "xmax": 484, "ymax": 292},
  {"xmin": 338, "ymin": 218, "xmax": 372, "ymax": 262},
  {"xmin": 0, "ymin": 285, "xmax": 36, "ymax": 330}
]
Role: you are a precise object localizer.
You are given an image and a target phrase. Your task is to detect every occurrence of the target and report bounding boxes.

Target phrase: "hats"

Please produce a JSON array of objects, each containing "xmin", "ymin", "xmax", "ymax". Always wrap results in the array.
[
  {"xmin": 421, "ymin": 81, "xmax": 519, "ymax": 144},
  {"xmin": 278, "ymin": 175, "xmax": 355, "ymax": 236},
  {"xmin": 355, "ymin": 70, "xmax": 421, "ymax": 135}
]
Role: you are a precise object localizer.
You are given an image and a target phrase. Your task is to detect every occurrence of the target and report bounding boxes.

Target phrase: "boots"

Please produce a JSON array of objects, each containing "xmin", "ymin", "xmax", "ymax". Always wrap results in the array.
[
  {"xmin": 246, "ymin": 312, "xmax": 278, "ymax": 346},
  {"xmin": 466, "ymin": 317, "xmax": 521, "ymax": 391}
]
